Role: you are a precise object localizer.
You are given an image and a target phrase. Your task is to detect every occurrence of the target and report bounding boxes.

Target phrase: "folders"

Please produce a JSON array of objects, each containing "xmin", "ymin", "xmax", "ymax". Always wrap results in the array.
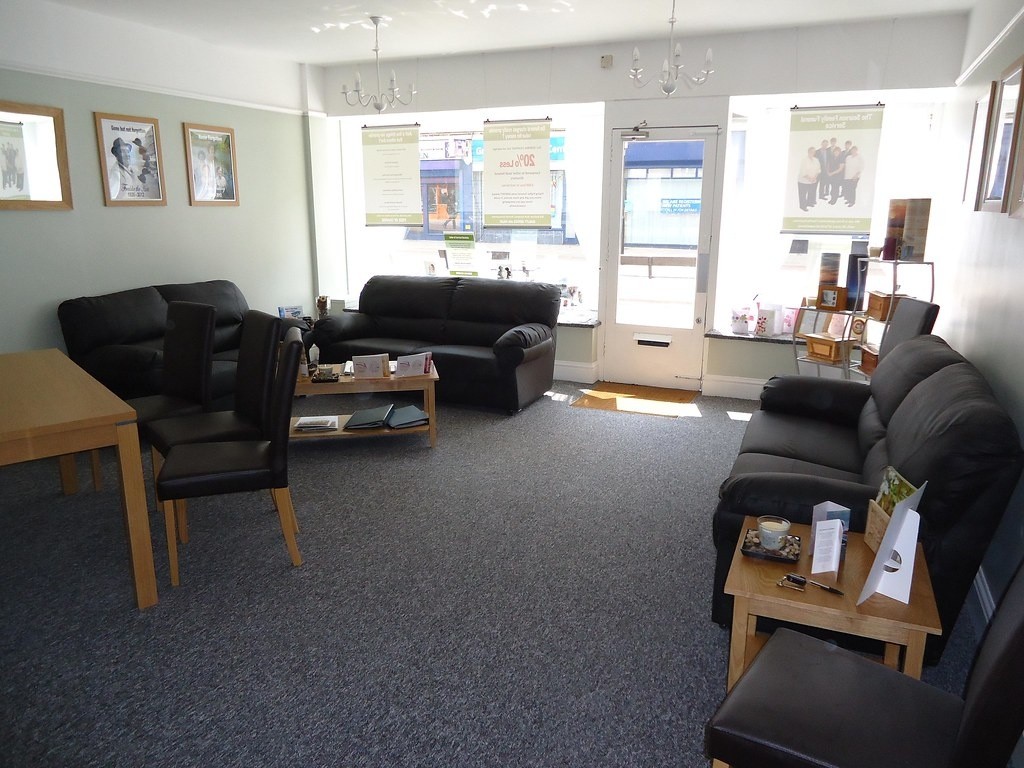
[
  {"xmin": 386, "ymin": 405, "xmax": 429, "ymax": 429},
  {"xmin": 343, "ymin": 403, "xmax": 394, "ymax": 430}
]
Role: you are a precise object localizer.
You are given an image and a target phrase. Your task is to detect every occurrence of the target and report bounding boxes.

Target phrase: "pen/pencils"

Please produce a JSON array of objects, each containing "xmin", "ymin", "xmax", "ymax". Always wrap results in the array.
[{"xmin": 806, "ymin": 579, "xmax": 845, "ymax": 596}]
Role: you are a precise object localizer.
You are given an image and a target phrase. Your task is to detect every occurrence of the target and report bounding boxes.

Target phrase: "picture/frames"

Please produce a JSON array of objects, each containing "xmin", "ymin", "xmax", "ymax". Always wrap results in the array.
[
  {"xmin": 93, "ymin": 112, "xmax": 167, "ymax": 206},
  {"xmin": 183, "ymin": 122, "xmax": 240, "ymax": 206}
]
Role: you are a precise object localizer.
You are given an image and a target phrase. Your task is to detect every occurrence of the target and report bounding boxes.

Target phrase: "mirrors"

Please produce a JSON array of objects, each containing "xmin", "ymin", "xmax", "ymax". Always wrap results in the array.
[
  {"xmin": 962, "ymin": 80, "xmax": 997, "ymax": 211},
  {"xmin": 982, "ymin": 54, "xmax": 1024, "ymax": 212},
  {"xmin": 0, "ymin": 100, "xmax": 73, "ymax": 211}
]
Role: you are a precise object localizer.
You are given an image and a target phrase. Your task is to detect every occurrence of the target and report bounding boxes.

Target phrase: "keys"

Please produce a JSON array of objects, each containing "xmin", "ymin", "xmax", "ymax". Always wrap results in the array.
[{"xmin": 786, "ymin": 572, "xmax": 807, "ymax": 586}]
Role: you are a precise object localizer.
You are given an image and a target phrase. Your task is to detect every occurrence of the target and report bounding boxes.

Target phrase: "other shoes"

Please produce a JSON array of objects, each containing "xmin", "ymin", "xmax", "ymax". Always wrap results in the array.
[
  {"xmin": 849, "ymin": 203, "xmax": 854, "ymax": 207},
  {"xmin": 807, "ymin": 193, "xmax": 849, "ymax": 207},
  {"xmin": 803, "ymin": 208, "xmax": 808, "ymax": 211}
]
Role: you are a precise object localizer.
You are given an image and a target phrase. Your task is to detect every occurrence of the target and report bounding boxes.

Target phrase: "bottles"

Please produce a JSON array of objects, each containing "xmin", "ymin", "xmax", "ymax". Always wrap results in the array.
[
  {"xmin": 296, "ymin": 346, "xmax": 309, "ymax": 382},
  {"xmin": 309, "ymin": 343, "xmax": 319, "ymax": 366}
]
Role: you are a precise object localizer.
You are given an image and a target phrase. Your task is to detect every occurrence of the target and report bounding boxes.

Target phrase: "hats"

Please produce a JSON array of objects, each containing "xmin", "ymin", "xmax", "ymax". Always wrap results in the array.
[{"xmin": 111, "ymin": 138, "xmax": 132, "ymax": 156}]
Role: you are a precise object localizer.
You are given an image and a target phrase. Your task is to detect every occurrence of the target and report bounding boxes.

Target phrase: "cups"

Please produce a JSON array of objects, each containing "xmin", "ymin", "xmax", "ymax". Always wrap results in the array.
[
  {"xmin": 896, "ymin": 245, "xmax": 915, "ymax": 261},
  {"xmin": 757, "ymin": 515, "xmax": 791, "ymax": 550},
  {"xmin": 278, "ymin": 307, "xmax": 285, "ymax": 319},
  {"xmin": 318, "ymin": 364, "xmax": 333, "ymax": 376},
  {"xmin": 883, "ymin": 238, "xmax": 896, "ymax": 260},
  {"xmin": 731, "ymin": 305, "xmax": 798, "ymax": 337}
]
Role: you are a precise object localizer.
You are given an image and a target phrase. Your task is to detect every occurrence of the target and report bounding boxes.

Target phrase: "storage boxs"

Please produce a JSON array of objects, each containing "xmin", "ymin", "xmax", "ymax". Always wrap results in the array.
[{"xmin": 794, "ymin": 285, "xmax": 909, "ymax": 380}]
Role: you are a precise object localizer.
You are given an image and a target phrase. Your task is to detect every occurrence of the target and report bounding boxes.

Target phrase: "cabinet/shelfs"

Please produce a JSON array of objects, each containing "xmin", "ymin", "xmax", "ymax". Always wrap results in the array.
[{"xmin": 792, "ymin": 259, "xmax": 934, "ymax": 386}]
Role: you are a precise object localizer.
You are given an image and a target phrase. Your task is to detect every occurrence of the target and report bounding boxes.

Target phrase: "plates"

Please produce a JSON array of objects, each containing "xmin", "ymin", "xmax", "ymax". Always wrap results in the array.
[
  {"xmin": 311, "ymin": 373, "xmax": 339, "ymax": 383},
  {"xmin": 740, "ymin": 528, "xmax": 801, "ymax": 563}
]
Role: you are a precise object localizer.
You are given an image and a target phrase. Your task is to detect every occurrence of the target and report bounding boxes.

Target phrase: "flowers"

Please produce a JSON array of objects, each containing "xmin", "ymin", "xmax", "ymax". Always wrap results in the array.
[{"xmin": 317, "ymin": 295, "xmax": 327, "ymax": 315}]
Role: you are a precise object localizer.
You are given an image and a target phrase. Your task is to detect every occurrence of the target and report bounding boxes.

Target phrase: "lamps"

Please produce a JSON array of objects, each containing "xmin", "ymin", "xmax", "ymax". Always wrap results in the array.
[
  {"xmin": 626, "ymin": 0, "xmax": 718, "ymax": 99},
  {"xmin": 340, "ymin": 16, "xmax": 418, "ymax": 114}
]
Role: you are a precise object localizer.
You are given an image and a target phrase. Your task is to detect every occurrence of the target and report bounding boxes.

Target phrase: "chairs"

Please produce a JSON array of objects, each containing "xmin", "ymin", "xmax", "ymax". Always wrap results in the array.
[
  {"xmin": 704, "ymin": 562, "xmax": 1024, "ymax": 768},
  {"xmin": 877, "ymin": 298, "xmax": 940, "ymax": 365},
  {"xmin": 126, "ymin": 302, "xmax": 302, "ymax": 585}
]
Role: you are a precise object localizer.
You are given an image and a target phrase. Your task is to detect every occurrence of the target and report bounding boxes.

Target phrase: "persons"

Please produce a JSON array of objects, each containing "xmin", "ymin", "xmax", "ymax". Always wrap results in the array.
[
  {"xmin": 798, "ymin": 138, "xmax": 863, "ymax": 211},
  {"xmin": 108, "ymin": 136, "xmax": 143, "ymax": 199},
  {"xmin": 0, "ymin": 142, "xmax": 24, "ymax": 192},
  {"xmin": 198, "ymin": 145, "xmax": 226, "ymax": 197},
  {"xmin": 443, "ymin": 190, "xmax": 456, "ymax": 229}
]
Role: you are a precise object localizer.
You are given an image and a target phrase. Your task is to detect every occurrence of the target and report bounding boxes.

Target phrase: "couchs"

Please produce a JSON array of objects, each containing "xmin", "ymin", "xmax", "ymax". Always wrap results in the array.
[
  {"xmin": 313, "ymin": 275, "xmax": 561, "ymax": 414},
  {"xmin": 711, "ymin": 331, "xmax": 1024, "ymax": 670},
  {"xmin": 57, "ymin": 280, "xmax": 312, "ymax": 438}
]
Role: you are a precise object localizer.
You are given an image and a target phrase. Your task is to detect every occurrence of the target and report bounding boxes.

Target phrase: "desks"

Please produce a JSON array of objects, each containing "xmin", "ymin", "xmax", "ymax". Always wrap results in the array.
[
  {"xmin": 0, "ymin": 348, "xmax": 158, "ymax": 608},
  {"xmin": 724, "ymin": 516, "xmax": 943, "ymax": 692},
  {"xmin": 288, "ymin": 364, "xmax": 439, "ymax": 449}
]
